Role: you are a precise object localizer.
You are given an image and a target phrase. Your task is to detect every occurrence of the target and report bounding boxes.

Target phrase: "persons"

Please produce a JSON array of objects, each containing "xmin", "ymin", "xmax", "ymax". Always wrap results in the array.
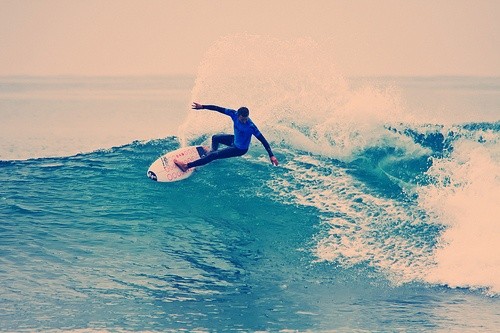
[{"xmin": 172, "ymin": 100, "xmax": 279, "ymax": 172}]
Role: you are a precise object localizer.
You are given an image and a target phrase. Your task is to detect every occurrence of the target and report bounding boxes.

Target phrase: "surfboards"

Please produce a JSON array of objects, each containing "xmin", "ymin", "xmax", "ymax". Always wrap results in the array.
[{"xmin": 147, "ymin": 145, "xmax": 210, "ymax": 182}]
